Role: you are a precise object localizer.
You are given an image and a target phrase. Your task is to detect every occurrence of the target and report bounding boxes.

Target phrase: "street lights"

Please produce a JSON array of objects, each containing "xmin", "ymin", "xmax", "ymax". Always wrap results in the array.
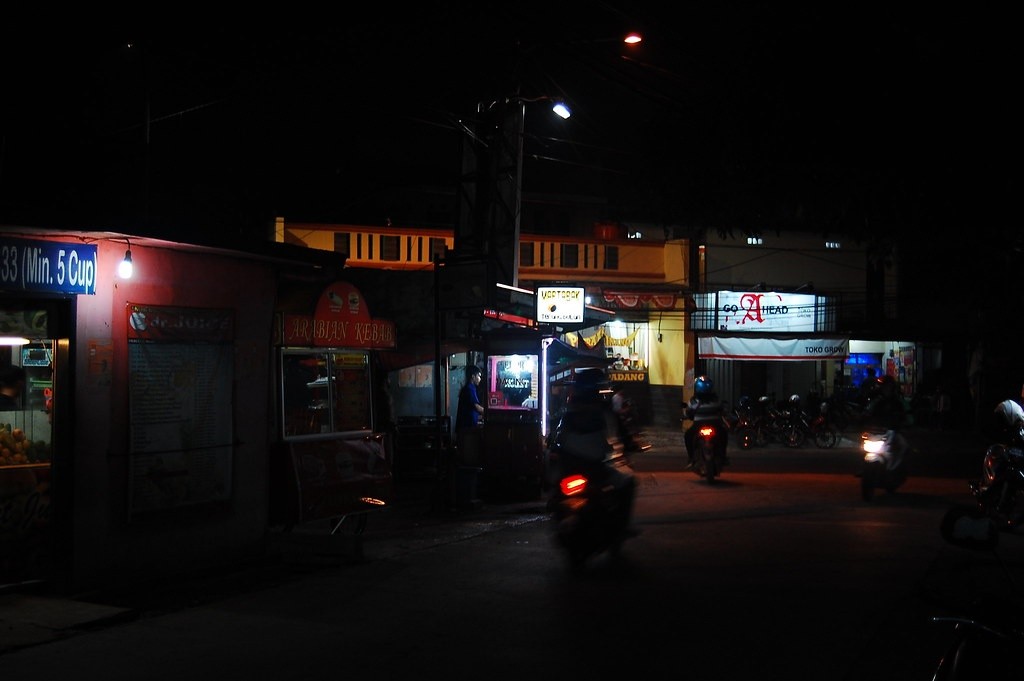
[{"xmin": 493, "ymin": 96, "xmax": 570, "ymax": 306}]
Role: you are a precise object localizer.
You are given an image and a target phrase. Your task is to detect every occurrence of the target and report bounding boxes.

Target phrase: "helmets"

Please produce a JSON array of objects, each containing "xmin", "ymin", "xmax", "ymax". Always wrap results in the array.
[
  {"xmin": 694, "ymin": 376, "xmax": 713, "ymax": 394},
  {"xmin": 865, "ymin": 365, "xmax": 878, "ymax": 375},
  {"xmin": 820, "ymin": 402, "xmax": 827, "ymax": 412},
  {"xmin": 788, "ymin": 394, "xmax": 800, "ymax": 402},
  {"xmin": 759, "ymin": 396, "xmax": 768, "ymax": 402}
]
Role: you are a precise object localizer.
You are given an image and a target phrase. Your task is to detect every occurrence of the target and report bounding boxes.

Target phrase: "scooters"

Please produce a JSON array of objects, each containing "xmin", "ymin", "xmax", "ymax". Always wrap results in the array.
[
  {"xmin": 984, "ymin": 410, "xmax": 1024, "ymax": 531},
  {"xmin": 678, "ymin": 400, "xmax": 731, "ymax": 484},
  {"xmin": 544, "ymin": 435, "xmax": 651, "ymax": 552},
  {"xmin": 855, "ymin": 418, "xmax": 915, "ymax": 503}
]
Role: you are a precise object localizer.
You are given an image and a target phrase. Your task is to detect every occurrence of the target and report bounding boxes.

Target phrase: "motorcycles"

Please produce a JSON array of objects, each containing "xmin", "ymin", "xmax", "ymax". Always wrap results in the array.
[{"xmin": 733, "ymin": 396, "xmax": 836, "ymax": 450}]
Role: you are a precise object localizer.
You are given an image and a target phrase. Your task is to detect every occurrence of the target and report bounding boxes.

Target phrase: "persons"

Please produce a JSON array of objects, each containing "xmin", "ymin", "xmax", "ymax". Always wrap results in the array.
[
  {"xmin": 455, "ymin": 364, "xmax": 486, "ymax": 435},
  {"xmin": 550, "ymin": 368, "xmax": 640, "ymax": 551},
  {"xmin": 682, "ymin": 377, "xmax": 728, "ymax": 473}
]
